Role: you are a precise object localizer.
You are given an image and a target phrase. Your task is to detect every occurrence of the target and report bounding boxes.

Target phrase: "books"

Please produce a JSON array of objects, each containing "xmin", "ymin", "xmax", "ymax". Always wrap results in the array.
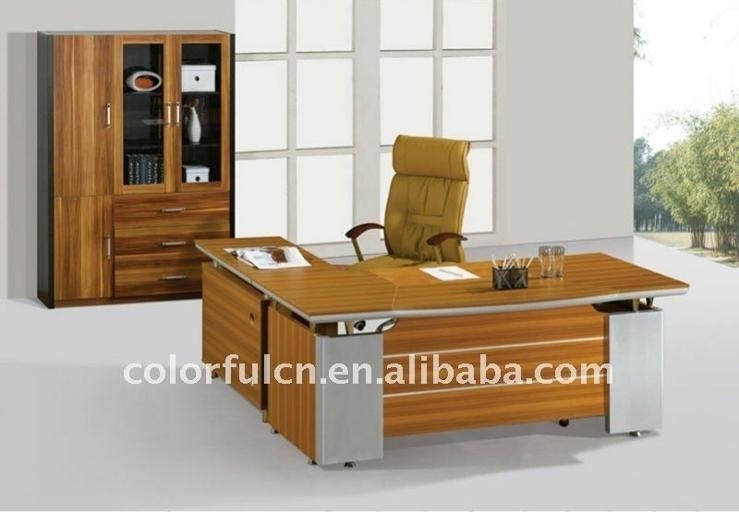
[
  {"xmin": 224, "ymin": 245, "xmax": 312, "ymax": 270},
  {"xmin": 419, "ymin": 264, "xmax": 482, "ymax": 283},
  {"xmin": 125, "ymin": 152, "xmax": 164, "ymax": 184}
]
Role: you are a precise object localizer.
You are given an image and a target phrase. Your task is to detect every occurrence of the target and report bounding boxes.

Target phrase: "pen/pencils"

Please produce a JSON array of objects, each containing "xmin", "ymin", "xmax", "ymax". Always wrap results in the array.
[{"xmin": 491, "ymin": 253, "xmax": 535, "ymax": 288}]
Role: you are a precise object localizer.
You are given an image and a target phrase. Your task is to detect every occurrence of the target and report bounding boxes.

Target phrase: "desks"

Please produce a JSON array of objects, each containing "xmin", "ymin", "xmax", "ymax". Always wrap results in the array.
[{"xmin": 194, "ymin": 237, "xmax": 691, "ymax": 468}]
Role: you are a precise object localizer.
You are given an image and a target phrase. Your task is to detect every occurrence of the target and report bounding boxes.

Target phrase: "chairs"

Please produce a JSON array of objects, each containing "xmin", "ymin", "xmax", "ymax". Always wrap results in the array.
[{"xmin": 345, "ymin": 135, "xmax": 471, "ymax": 268}]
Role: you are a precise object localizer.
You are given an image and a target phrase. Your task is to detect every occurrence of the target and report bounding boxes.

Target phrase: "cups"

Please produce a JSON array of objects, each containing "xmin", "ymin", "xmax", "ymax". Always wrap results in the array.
[{"xmin": 537, "ymin": 246, "xmax": 566, "ymax": 279}]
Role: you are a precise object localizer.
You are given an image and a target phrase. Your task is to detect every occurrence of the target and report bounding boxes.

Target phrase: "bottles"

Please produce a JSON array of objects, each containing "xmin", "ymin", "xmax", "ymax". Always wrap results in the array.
[{"xmin": 186, "ymin": 106, "xmax": 201, "ymax": 143}]
